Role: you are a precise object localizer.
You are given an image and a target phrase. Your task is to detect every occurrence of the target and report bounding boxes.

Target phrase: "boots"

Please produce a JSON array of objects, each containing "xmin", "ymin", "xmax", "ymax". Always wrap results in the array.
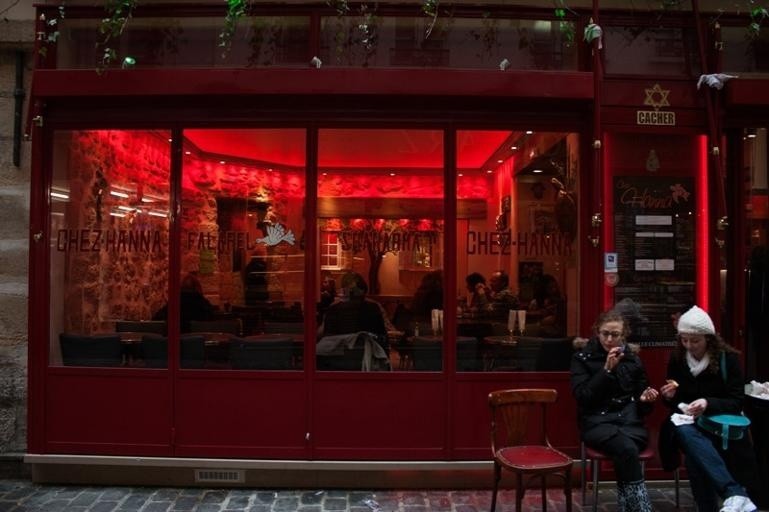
[
  {"xmin": 617, "ymin": 478, "xmax": 629, "ymax": 512},
  {"xmin": 623, "ymin": 479, "xmax": 654, "ymax": 512}
]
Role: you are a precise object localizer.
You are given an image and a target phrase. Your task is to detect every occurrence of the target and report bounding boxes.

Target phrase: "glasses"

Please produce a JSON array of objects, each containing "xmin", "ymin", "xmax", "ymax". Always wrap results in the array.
[{"xmin": 598, "ymin": 331, "xmax": 625, "ymax": 338}]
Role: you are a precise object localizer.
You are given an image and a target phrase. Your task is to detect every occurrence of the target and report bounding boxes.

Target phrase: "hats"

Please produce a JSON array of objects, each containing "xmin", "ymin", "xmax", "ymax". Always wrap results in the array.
[{"xmin": 677, "ymin": 305, "xmax": 716, "ymax": 337}]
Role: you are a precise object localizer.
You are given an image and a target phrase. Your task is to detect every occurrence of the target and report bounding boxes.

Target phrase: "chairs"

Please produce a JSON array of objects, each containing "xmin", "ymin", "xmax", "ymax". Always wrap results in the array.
[
  {"xmin": 487, "ymin": 386, "xmax": 576, "ymax": 512},
  {"xmin": 55, "ymin": 297, "xmax": 582, "ymax": 374},
  {"xmin": 673, "ymin": 448, "xmax": 691, "ymax": 511},
  {"xmin": 578, "ymin": 419, "xmax": 657, "ymax": 511}
]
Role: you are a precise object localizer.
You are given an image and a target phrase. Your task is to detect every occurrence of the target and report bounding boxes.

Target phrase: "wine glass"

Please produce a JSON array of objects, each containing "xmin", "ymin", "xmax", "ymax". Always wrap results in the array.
[
  {"xmin": 431, "ymin": 310, "xmax": 444, "ymax": 337},
  {"xmin": 506, "ymin": 310, "xmax": 527, "ymax": 340}
]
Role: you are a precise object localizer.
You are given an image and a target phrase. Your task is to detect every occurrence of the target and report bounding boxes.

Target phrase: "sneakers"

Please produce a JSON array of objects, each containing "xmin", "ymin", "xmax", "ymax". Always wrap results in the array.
[{"xmin": 719, "ymin": 495, "xmax": 758, "ymax": 512}]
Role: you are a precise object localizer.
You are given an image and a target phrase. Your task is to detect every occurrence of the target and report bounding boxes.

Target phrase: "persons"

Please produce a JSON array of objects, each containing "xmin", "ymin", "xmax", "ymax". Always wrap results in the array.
[
  {"xmin": 572, "ymin": 308, "xmax": 661, "ymax": 512},
  {"xmin": 147, "ymin": 257, "xmax": 569, "ymax": 375},
  {"xmin": 658, "ymin": 304, "xmax": 769, "ymax": 512}
]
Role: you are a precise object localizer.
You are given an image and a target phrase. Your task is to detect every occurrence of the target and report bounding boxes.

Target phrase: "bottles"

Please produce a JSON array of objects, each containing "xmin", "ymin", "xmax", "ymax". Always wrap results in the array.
[{"xmin": 414, "ymin": 322, "xmax": 420, "ymax": 336}]
